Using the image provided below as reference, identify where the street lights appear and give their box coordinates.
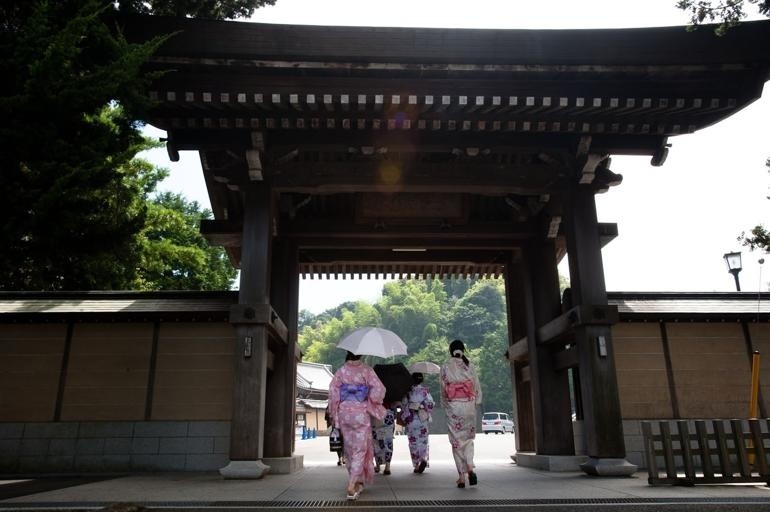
[723,251,743,291]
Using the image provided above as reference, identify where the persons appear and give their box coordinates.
[401,372,436,474]
[325,350,397,500]
[439,339,483,488]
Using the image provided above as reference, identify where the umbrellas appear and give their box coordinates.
[407,361,441,373]
[337,326,408,359]
[373,362,413,408]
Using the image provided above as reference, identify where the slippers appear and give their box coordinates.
[457,470,478,488]
[346,482,365,500]
[374,465,391,475]
[337,460,346,465]
[413,459,427,474]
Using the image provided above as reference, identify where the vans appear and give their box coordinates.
[481,412,515,434]
